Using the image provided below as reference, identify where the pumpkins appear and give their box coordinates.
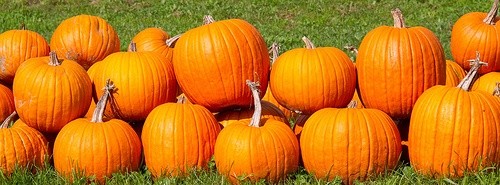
[0,0,500,185]
